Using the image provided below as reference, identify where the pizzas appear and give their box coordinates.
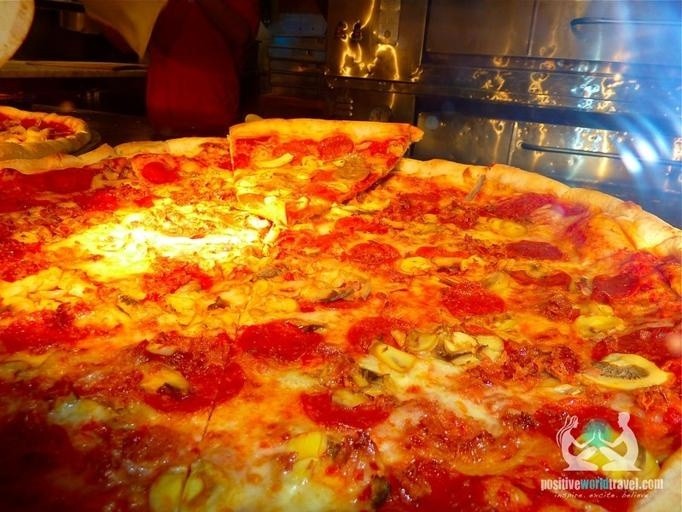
[0,117,682,511]
[0,105,92,162]
